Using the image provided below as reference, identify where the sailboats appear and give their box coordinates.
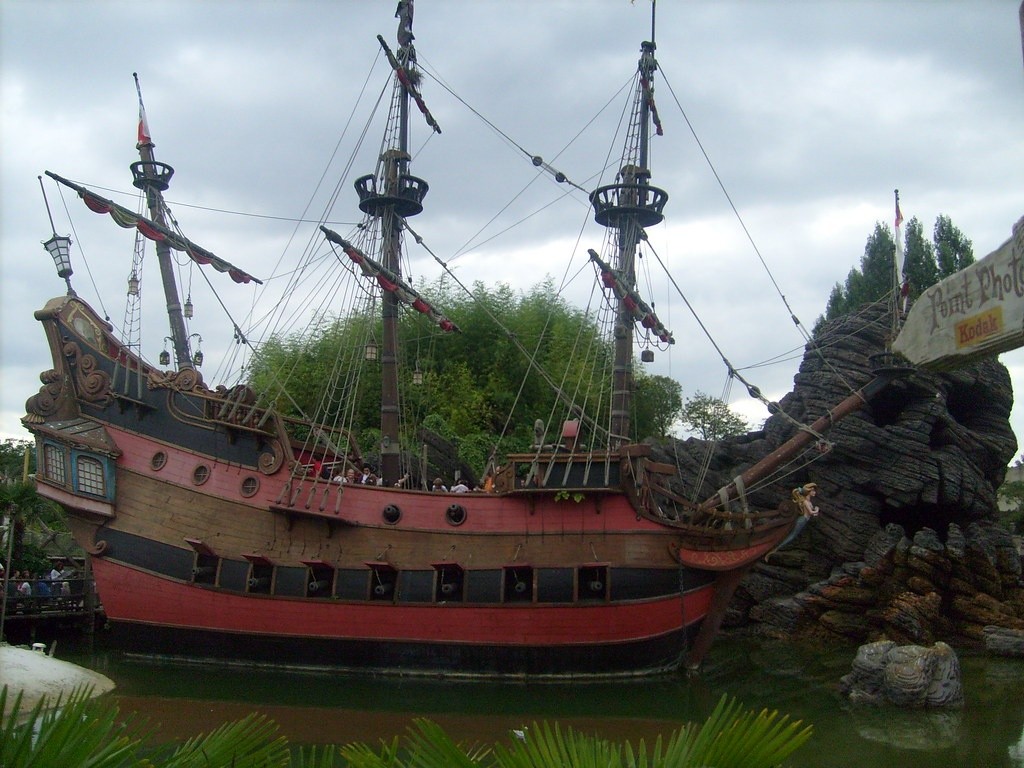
[18,1,917,690]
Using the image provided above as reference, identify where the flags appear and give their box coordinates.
[137,83,152,142]
[894,206,910,315]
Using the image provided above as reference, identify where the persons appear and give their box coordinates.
[309,454,324,477]
[333,467,347,482]
[346,468,358,484]
[8,561,72,604]
[432,478,448,492]
[357,462,377,485]
[450,478,471,493]
[765,483,820,562]
[485,473,493,491]
